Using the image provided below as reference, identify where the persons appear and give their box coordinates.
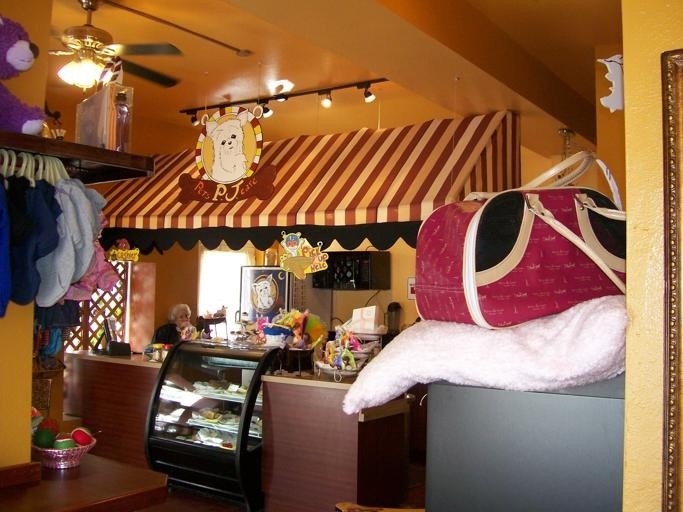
[150,303,195,347]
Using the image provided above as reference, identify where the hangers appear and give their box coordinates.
[1,147,72,193]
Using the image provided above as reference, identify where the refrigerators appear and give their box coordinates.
[235,264,332,335]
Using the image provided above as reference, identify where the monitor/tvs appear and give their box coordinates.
[269,348,314,375]
[90,318,113,353]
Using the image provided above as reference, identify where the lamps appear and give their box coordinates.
[179,73,385,126]
[57,52,114,90]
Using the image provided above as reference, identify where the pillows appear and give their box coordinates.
[341,290,641,418]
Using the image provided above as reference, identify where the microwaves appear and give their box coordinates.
[312,250,391,290]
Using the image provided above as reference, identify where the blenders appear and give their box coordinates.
[382,301,401,336]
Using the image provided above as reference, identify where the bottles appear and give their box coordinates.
[152,343,174,362]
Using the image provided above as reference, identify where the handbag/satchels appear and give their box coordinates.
[413,186,627,331]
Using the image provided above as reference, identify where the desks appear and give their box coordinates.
[0,442,170,511]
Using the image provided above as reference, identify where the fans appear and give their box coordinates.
[50,0,184,90]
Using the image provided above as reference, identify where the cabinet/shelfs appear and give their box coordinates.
[313,251,390,290]
[143,339,288,512]
